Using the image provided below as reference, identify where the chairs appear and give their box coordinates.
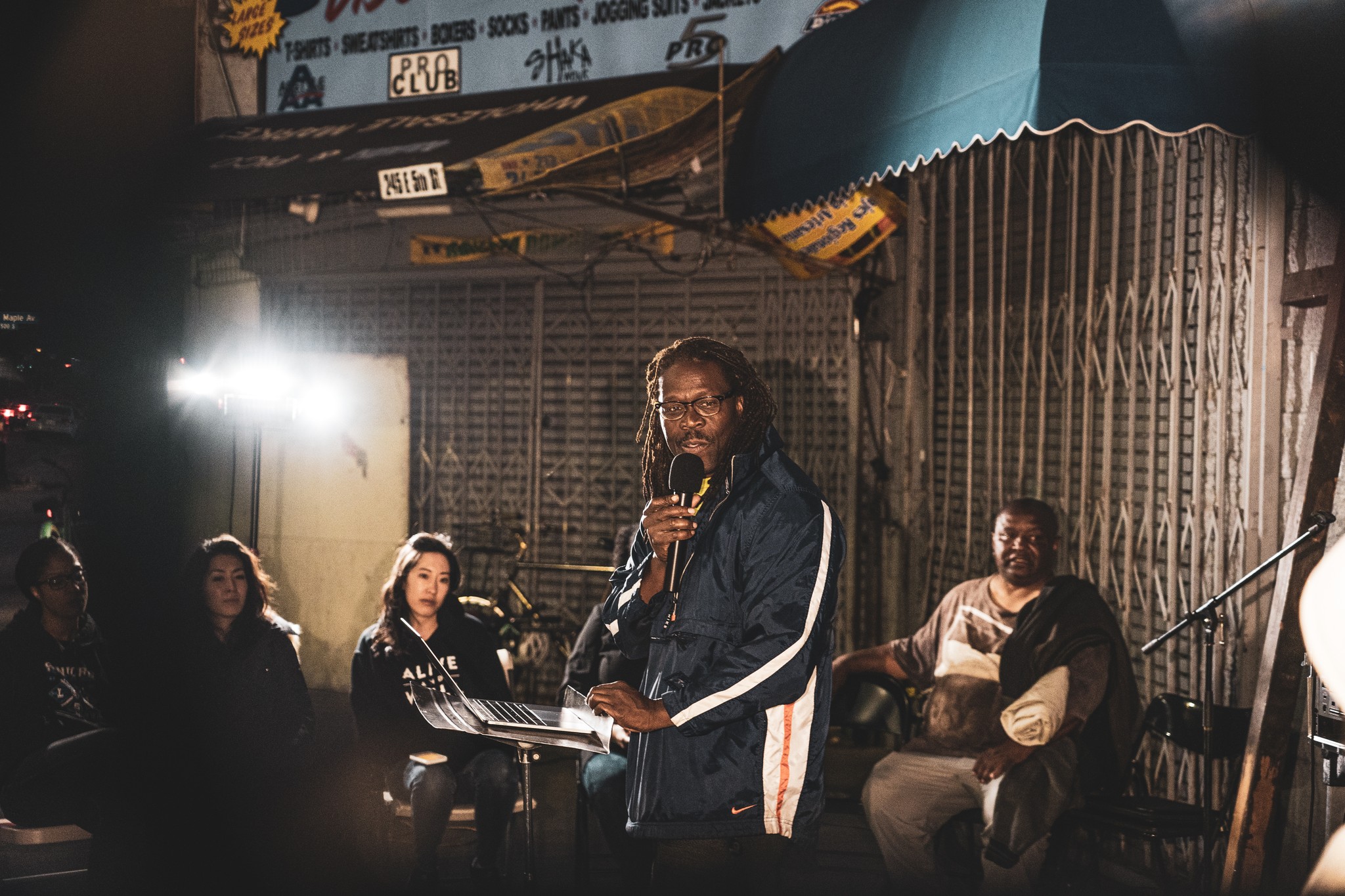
[1032,687,1262,896]
[732,671,915,896]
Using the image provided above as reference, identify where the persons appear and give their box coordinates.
[0,536,151,896]
[351,533,517,896]
[833,498,1110,896]
[558,522,658,896]
[173,533,318,896]
[584,337,847,896]
[1298,529,1345,896]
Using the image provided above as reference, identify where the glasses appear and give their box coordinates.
[42,569,86,591]
[655,389,737,420]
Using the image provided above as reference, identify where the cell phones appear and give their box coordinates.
[409,750,448,765]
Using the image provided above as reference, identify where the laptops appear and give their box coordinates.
[400,617,594,734]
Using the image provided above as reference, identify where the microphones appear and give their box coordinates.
[662,453,704,593]
